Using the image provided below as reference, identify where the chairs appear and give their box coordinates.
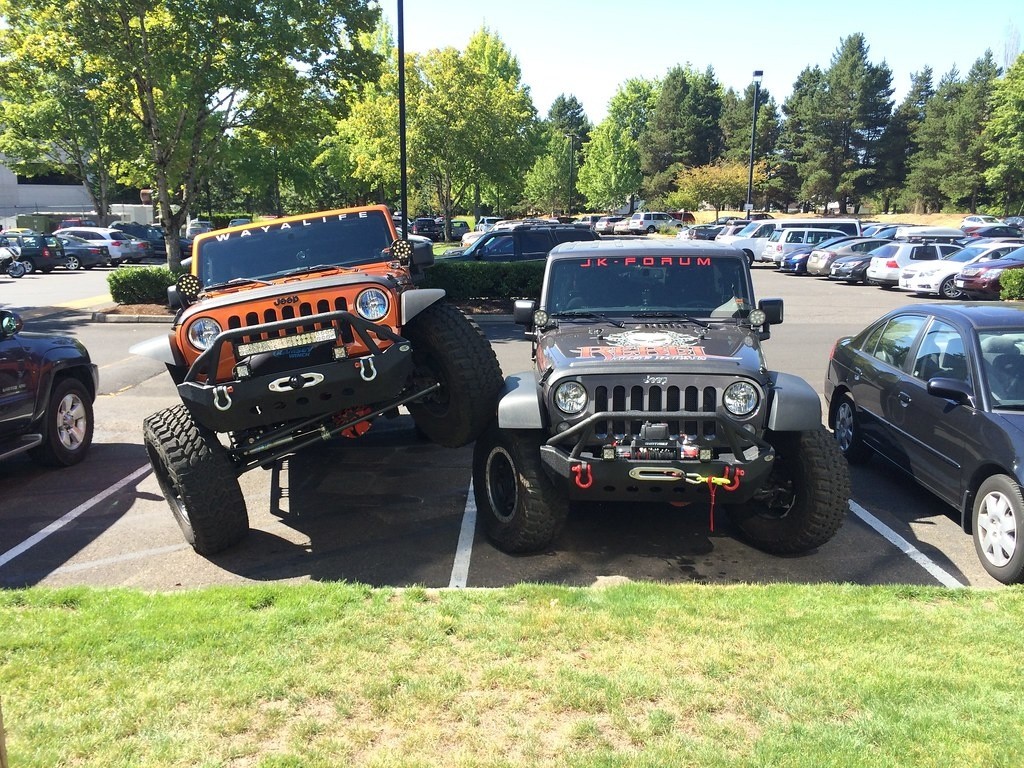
[812,236,820,244]
[991,252,1001,260]
[674,270,721,309]
[980,337,1020,372]
[793,238,802,243]
[931,338,1006,405]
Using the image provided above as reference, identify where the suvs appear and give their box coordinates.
[473,236,850,553]
[140,204,506,556]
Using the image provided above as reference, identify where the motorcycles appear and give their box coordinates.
[0,242,25,278]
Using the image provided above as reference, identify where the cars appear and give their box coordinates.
[825,304,1024,587]
[1,218,250,272]
[689,215,1024,299]
[392,210,696,255]
[0,310,99,469]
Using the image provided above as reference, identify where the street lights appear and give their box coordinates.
[746,69,764,203]
